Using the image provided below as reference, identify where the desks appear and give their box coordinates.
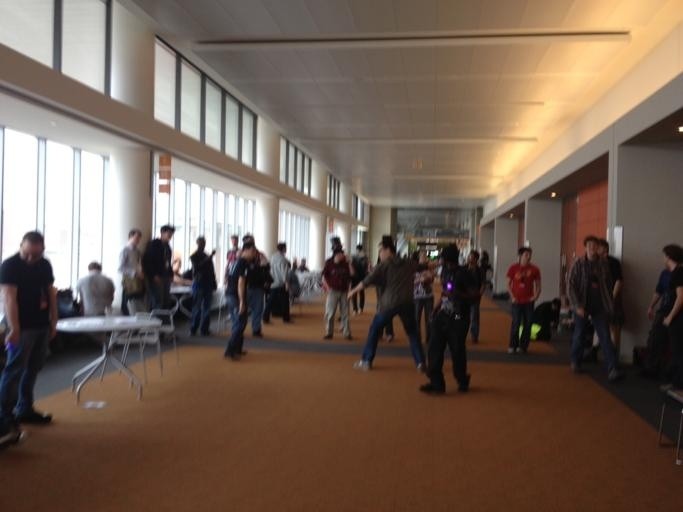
[295,271,324,297]
[169,287,193,319]
[55,316,163,404]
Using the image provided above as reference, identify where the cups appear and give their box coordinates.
[104,305,110,318]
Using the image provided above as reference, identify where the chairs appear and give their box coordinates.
[106,306,164,385]
[139,295,179,354]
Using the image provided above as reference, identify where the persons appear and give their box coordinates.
[75,263,115,316]
[645,244,683,393]
[505,247,541,354]
[564,238,622,384]
[0,316,28,447]
[3,231,58,434]
[118,225,309,360]
[322,235,489,393]
[532,298,561,342]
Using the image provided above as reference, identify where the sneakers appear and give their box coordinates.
[17,413,51,424]
[323,309,479,394]
[507,346,525,355]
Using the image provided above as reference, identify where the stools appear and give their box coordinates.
[657,385,683,466]
